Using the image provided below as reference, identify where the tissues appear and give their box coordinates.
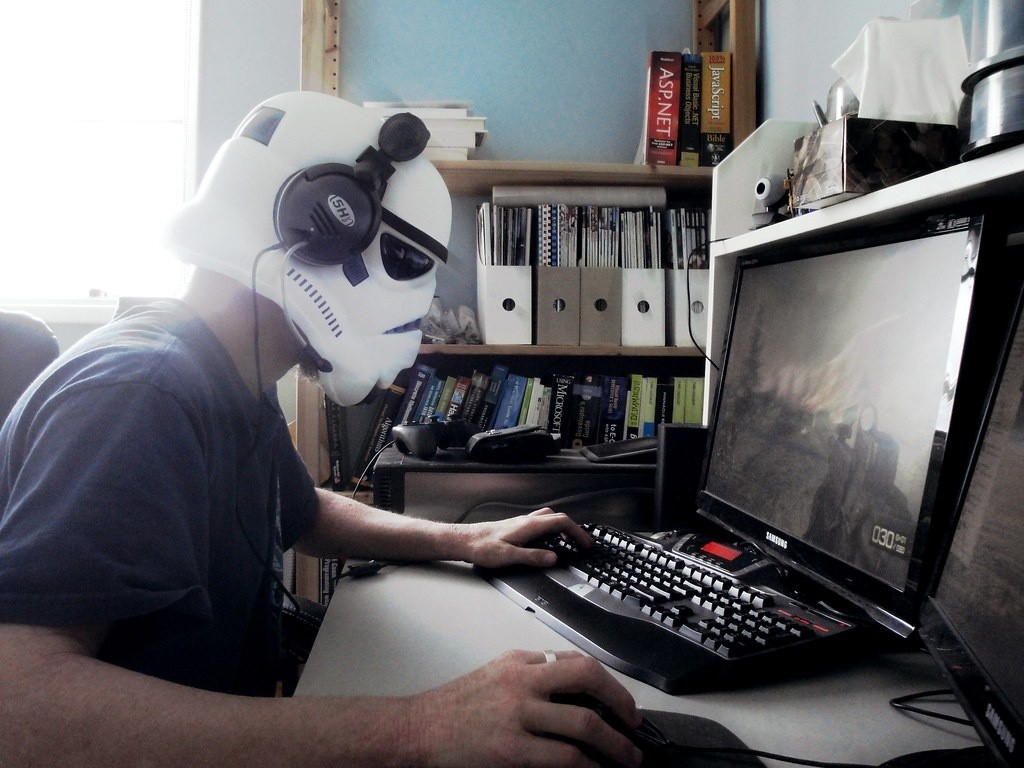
[793,15,969,211]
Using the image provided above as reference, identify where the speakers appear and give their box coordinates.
[654,422,710,547]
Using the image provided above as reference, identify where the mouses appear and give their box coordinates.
[530,682,670,768]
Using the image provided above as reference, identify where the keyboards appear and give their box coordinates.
[474,522,860,696]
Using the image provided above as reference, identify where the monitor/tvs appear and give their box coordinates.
[881,296,1024,768]
[701,168,1024,642]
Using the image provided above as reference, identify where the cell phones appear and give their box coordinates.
[581,436,658,461]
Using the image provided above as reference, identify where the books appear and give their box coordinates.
[323,361,706,495]
[475,200,711,267]
[317,555,341,608]
[644,49,732,169]
[358,95,487,161]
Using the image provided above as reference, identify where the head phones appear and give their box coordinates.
[273,113,432,267]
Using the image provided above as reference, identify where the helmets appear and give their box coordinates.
[174,91,451,406]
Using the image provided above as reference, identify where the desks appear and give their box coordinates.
[290,560,986,768]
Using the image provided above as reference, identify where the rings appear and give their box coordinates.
[541,650,558,665]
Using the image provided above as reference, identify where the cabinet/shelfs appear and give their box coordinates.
[294,0,756,606]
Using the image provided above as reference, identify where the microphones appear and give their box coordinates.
[281,241,335,374]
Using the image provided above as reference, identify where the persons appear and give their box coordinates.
[0,88,646,768]
